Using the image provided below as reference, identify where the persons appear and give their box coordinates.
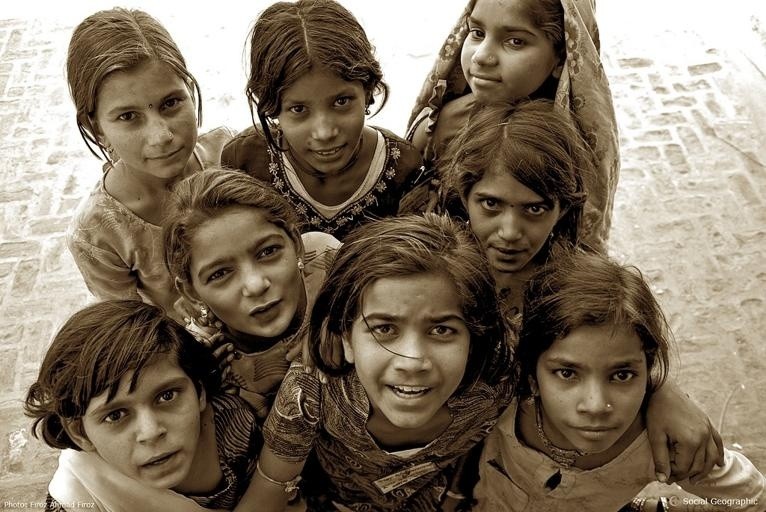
[435,93,601,383]
[463,235,766,510]
[169,0,431,329]
[63,4,288,388]
[229,209,729,511]
[20,295,321,512]
[398,0,624,264]
[159,167,354,430]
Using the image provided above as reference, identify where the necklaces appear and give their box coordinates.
[287,134,366,180]
[532,397,595,470]
